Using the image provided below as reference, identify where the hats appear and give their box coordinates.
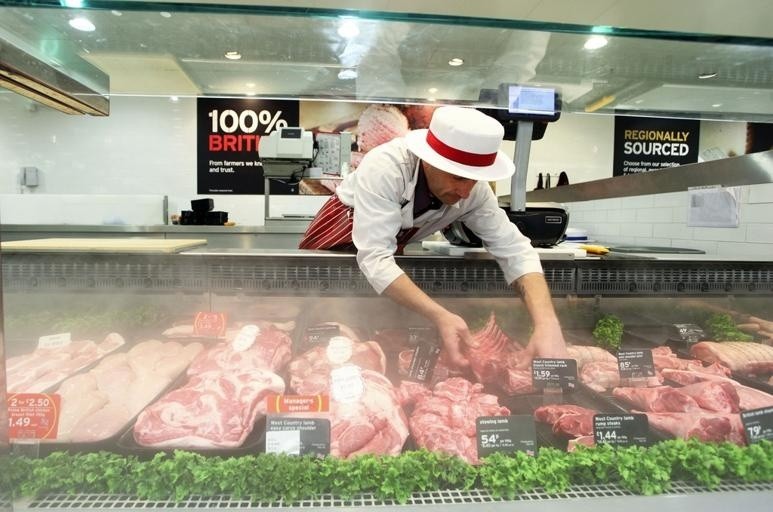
[401,105,516,182]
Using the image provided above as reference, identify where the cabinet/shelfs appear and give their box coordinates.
[0,246,773,512]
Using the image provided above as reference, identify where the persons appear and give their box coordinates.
[297,106,570,371]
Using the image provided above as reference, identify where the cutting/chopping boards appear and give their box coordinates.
[0,237,209,256]
